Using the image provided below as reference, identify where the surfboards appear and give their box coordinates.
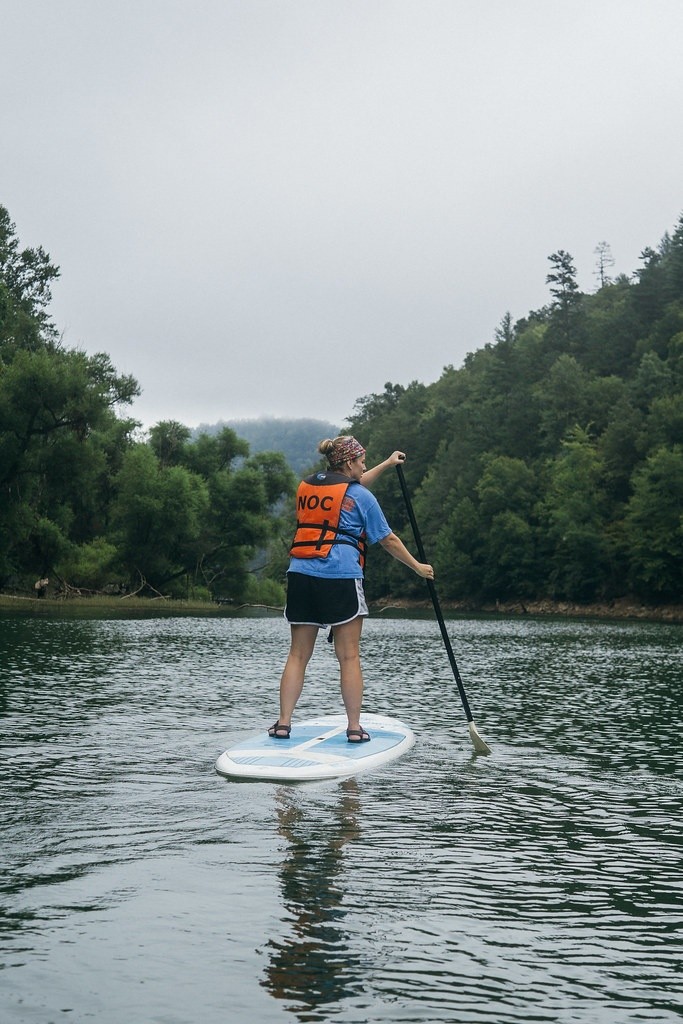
[212,710,418,782]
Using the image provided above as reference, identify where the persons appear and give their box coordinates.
[267,436,434,743]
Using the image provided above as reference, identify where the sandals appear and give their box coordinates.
[346,725,370,743]
[268,720,292,738]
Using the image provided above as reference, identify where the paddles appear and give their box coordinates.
[394,454,495,753]
